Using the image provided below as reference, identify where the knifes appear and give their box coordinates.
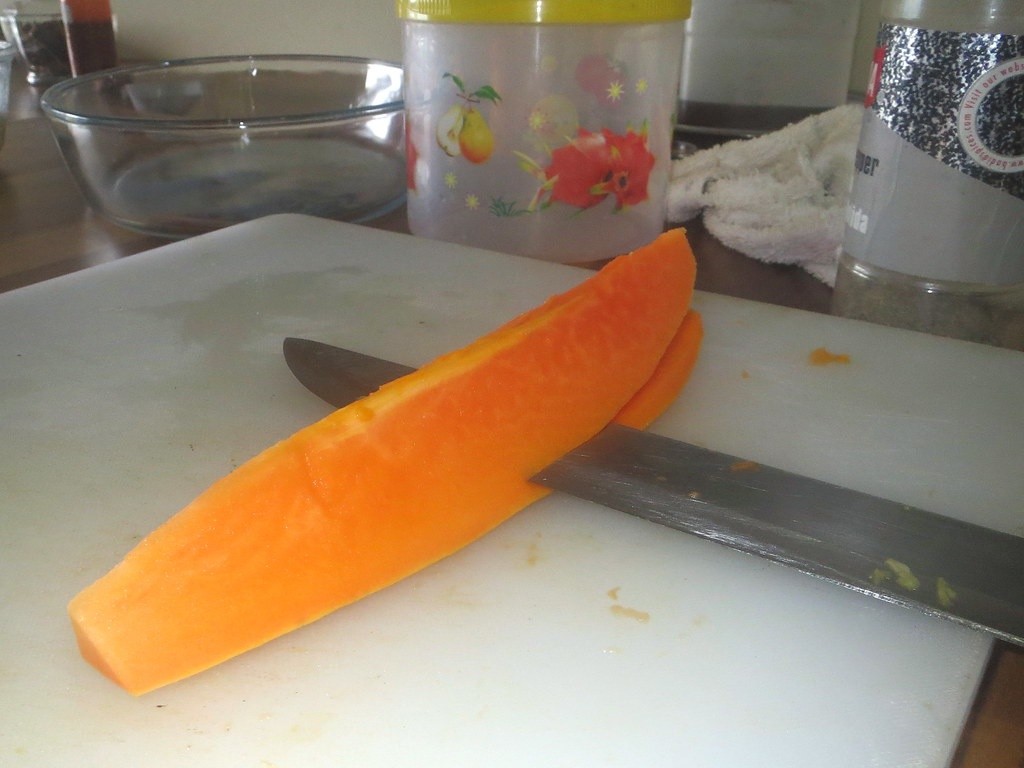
[284,337,1024,650]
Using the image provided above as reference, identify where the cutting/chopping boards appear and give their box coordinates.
[1,211,1024,768]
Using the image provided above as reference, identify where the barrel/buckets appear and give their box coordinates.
[399,0,694,264]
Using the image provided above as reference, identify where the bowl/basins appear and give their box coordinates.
[0,8,119,86]
[41,51,404,239]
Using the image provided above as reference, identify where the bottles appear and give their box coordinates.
[61,0,118,80]
[830,1,1024,346]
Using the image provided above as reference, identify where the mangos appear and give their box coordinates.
[69,224,702,699]
